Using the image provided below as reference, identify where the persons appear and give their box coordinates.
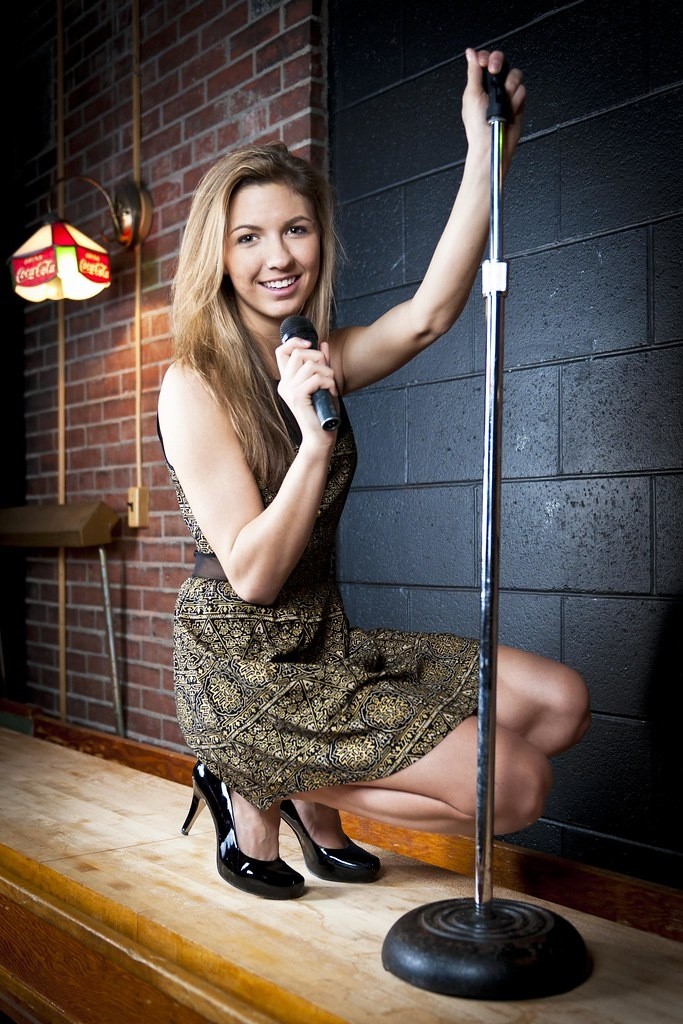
[155,42,591,901]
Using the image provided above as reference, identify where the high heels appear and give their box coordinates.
[280,799,381,883]
[182,760,305,900]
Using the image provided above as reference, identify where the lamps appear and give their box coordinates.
[12,174,154,302]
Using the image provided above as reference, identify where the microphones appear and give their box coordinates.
[280,315,340,432]
